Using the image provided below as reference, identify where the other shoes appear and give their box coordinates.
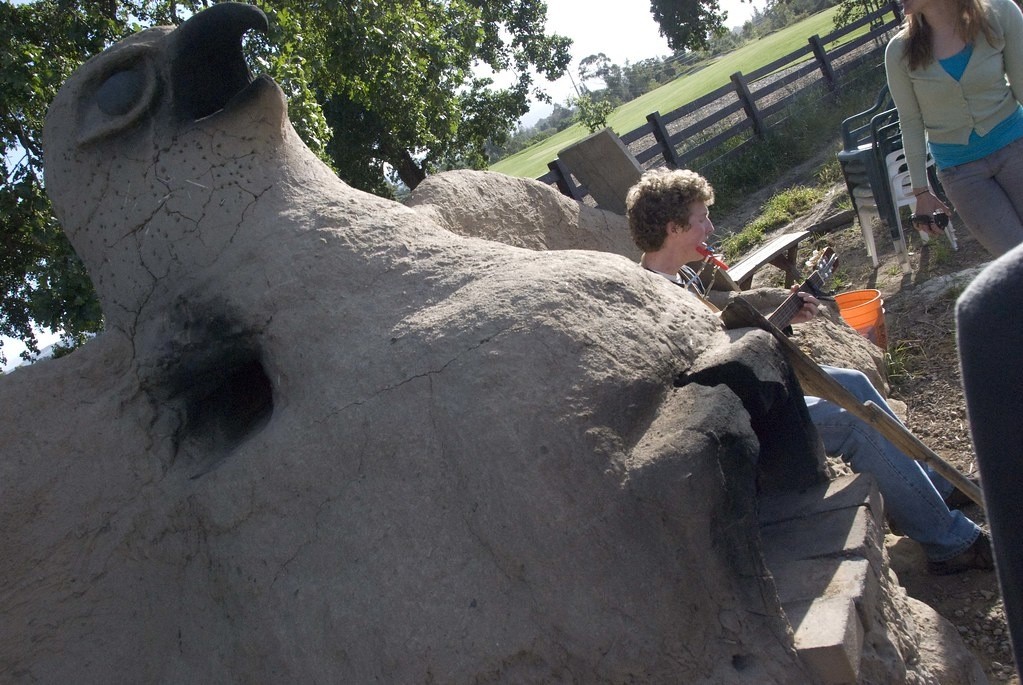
[942,476,982,512]
[931,530,996,573]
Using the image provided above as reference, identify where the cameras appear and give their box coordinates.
[933,212,949,230]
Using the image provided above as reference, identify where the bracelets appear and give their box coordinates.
[915,190,929,196]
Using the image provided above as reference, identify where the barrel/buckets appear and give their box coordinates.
[833,289,888,353]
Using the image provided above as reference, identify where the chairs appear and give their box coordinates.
[838,82,959,274]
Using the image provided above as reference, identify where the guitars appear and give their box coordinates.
[767,246,841,332]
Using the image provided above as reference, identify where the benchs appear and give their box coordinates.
[725,230,811,292]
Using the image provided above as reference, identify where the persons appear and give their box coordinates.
[885,0,1023,258]
[626,167,992,576]
